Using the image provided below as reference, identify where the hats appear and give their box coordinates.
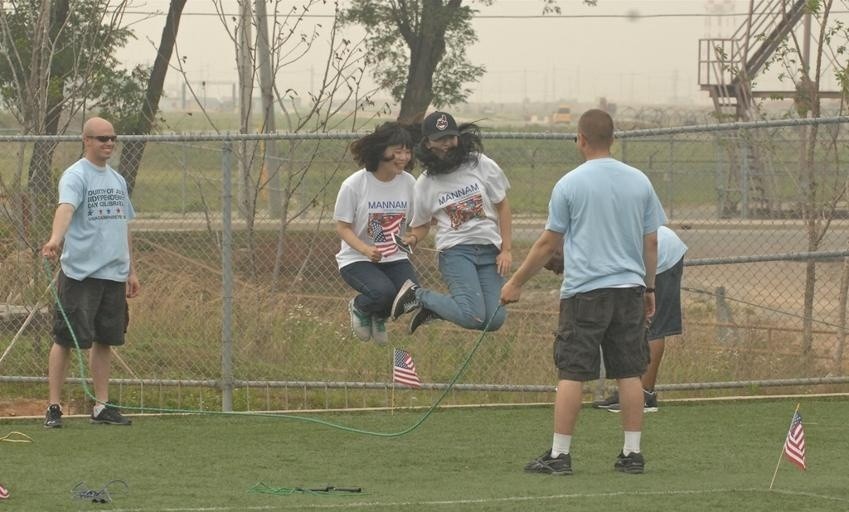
[421,111,461,140]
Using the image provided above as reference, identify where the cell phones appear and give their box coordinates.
[391,233,413,255]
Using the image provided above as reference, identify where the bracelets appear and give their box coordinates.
[644,287,656,293]
[407,234,418,245]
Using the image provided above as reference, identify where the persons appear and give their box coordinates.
[390,112,513,334]
[541,223,689,412]
[334,122,420,345]
[42,117,142,428]
[499,108,667,475]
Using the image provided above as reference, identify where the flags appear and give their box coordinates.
[370,216,402,257]
[394,347,421,390]
[783,409,808,470]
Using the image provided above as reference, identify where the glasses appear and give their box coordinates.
[87,136,116,142]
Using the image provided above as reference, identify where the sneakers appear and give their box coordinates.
[592,392,621,409]
[524,449,573,476]
[89,402,131,425]
[348,280,433,345]
[46,404,64,426]
[608,389,658,413]
[614,449,645,473]
